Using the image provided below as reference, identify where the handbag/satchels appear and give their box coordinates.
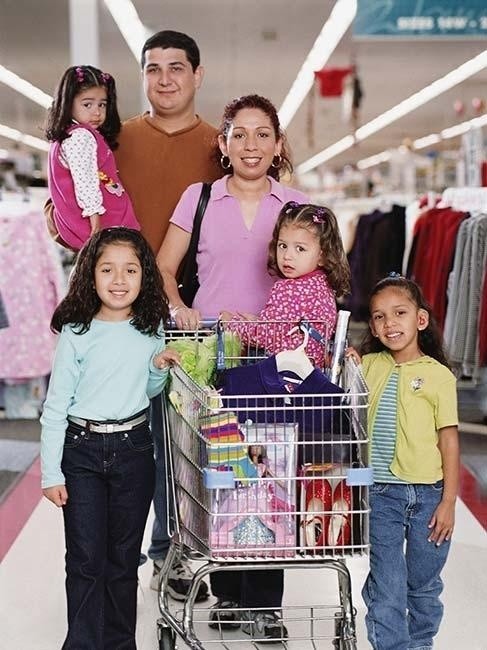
[174,245,200,310]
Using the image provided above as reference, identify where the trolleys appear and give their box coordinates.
[155,317,371,650]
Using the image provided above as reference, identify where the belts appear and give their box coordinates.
[67,412,149,436]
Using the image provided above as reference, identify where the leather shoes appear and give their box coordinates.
[303,475,333,551]
[325,478,357,546]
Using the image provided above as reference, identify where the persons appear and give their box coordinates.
[344,274,462,649]
[45,64,144,248]
[42,28,229,602]
[214,199,352,396]
[153,92,306,647]
[37,225,181,650]
[214,439,288,544]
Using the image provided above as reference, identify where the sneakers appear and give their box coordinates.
[149,555,210,603]
[207,598,244,630]
[240,610,289,644]
